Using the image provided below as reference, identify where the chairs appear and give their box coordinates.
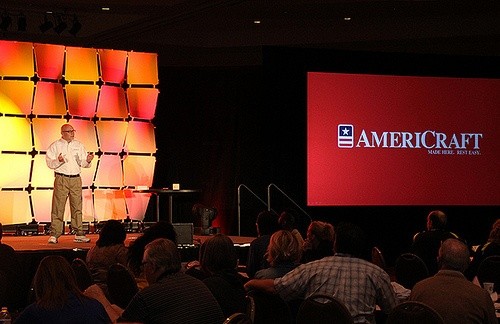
[73,246,500,324]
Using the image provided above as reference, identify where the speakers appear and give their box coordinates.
[171,223,194,244]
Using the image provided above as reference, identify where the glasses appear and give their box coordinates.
[62,130,76,134]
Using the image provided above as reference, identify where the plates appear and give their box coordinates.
[494,303,500,309]
[495,313,500,317]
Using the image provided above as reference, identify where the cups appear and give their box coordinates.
[173,184,179,190]
[483,283,493,293]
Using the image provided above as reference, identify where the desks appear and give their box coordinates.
[133,189,202,223]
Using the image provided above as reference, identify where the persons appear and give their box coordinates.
[0,209,500,324]
[45,123,94,243]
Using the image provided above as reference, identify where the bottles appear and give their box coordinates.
[0,307,11,324]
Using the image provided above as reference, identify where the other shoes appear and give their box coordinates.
[74,234,91,242]
[48,236,58,243]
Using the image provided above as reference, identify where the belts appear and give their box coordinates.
[55,172,80,178]
